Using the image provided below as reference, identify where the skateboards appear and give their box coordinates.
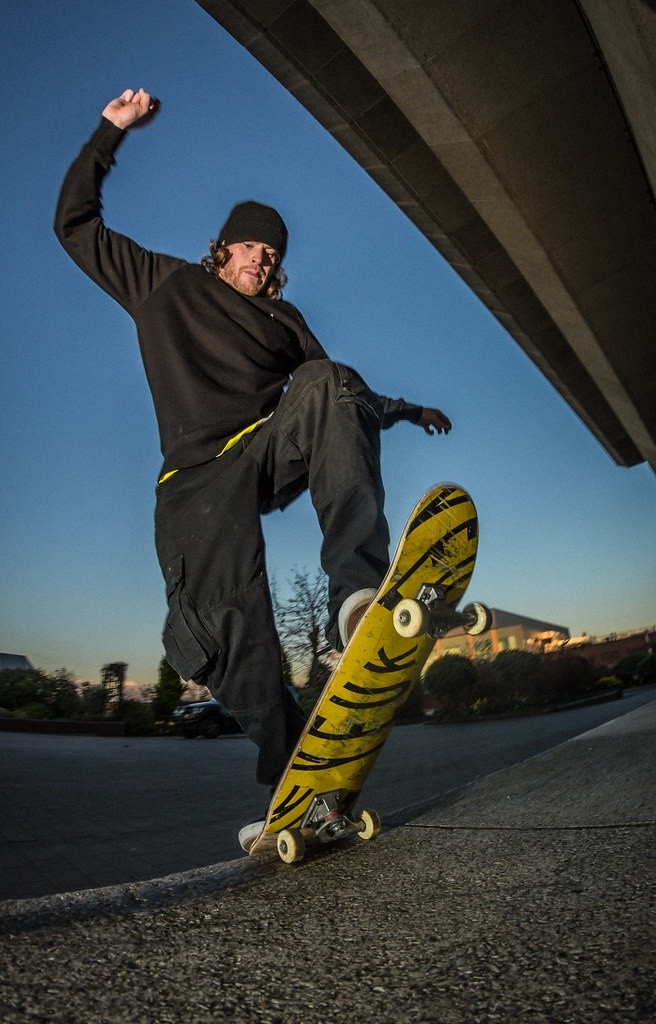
[248,481,494,865]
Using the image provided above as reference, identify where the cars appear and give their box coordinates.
[173,682,300,741]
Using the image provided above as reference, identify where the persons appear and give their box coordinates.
[54,85,452,854]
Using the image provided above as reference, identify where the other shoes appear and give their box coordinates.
[237,816,265,852]
[336,587,378,648]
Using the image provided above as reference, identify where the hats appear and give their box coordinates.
[216,201,288,264]
